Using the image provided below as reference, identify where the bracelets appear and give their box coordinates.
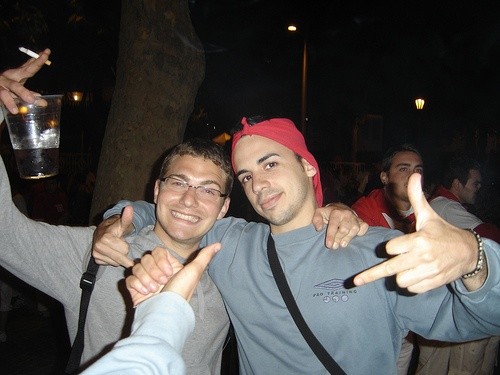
[462,228,484,279]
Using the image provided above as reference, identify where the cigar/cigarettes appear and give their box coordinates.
[19,47,51,66]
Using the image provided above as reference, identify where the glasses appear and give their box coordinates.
[160,177,228,202]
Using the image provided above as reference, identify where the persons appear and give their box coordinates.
[0,48,368,375]
[78,242,223,375]
[349,142,500,375]
[91,119,500,375]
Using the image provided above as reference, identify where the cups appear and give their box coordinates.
[2,94,62,179]
